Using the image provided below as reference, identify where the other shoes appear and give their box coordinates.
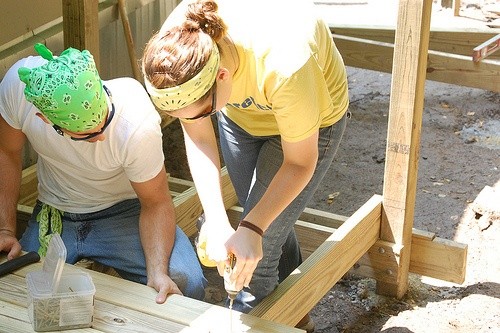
[297,320,315,333]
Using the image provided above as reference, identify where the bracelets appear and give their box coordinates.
[239,221,265,236]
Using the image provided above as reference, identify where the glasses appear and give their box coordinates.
[39,83,116,141]
[184,80,217,120]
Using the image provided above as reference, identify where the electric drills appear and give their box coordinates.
[195,214,244,331]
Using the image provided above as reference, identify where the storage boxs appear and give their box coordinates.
[25,231,95,332]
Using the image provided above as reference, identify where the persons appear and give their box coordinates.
[1,43,208,304]
[140,0,350,333]
